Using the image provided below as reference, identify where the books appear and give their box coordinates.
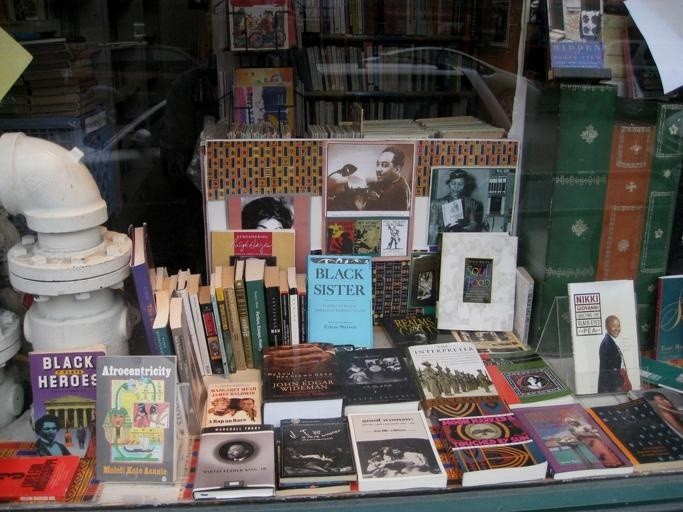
[0,0,683,506]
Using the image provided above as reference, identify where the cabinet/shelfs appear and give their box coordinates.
[208,0,306,139]
[292,1,490,122]
[0,350,682,512]
[0,0,155,221]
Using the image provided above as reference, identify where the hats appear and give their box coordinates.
[446,169,467,184]
